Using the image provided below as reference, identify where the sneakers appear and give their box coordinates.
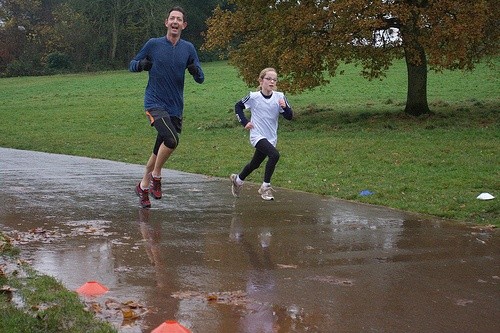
[135,182,151,208]
[147,172,161,199]
[258,185,277,201]
[230,174,244,198]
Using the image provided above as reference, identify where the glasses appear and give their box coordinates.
[264,78,277,82]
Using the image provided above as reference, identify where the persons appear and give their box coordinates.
[128,5,205,208]
[229,68,293,200]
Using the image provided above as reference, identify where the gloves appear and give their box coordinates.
[188,64,198,76]
[139,57,153,71]
